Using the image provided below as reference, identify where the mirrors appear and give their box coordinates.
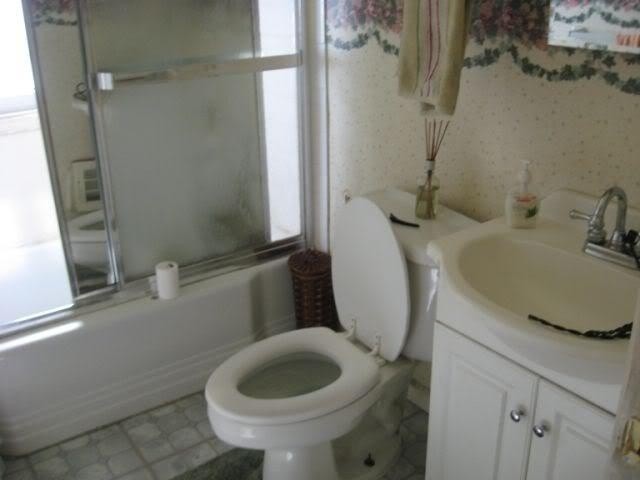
[20,0,120,304]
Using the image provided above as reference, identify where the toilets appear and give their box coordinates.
[202,187,482,480]
[67,208,109,270]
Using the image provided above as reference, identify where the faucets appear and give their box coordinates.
[585,185,629,248]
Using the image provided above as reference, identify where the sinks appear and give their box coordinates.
[424,187,640,387]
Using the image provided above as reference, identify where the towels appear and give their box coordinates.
[398,2,473,118]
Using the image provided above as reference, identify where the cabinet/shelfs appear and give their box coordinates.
[424,323,639,480]
